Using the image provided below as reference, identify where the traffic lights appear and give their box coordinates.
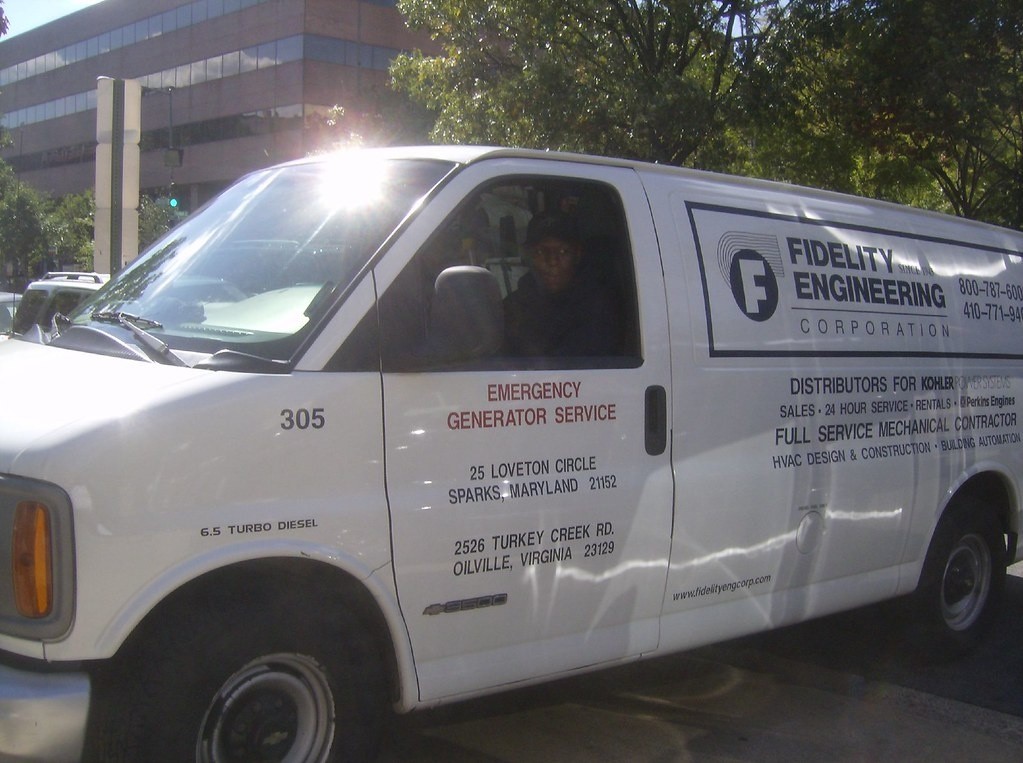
[168,182,181,208]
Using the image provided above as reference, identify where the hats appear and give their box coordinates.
[520,209,580,248]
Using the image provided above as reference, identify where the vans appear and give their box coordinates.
[1,140,1022,763]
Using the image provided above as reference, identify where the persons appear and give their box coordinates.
[491,197,624,359]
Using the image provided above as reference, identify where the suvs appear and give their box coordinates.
[0,267,114,346]
